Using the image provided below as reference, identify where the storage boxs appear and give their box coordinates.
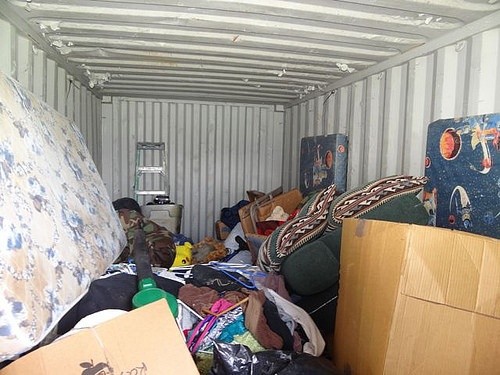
[0,297,200,375]
[333,218,500,375]
[141,204,183,234]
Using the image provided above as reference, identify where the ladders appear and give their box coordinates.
[132,141,172,202]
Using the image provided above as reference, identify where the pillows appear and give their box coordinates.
[258,183,337,274]
[325,174,430,235]
